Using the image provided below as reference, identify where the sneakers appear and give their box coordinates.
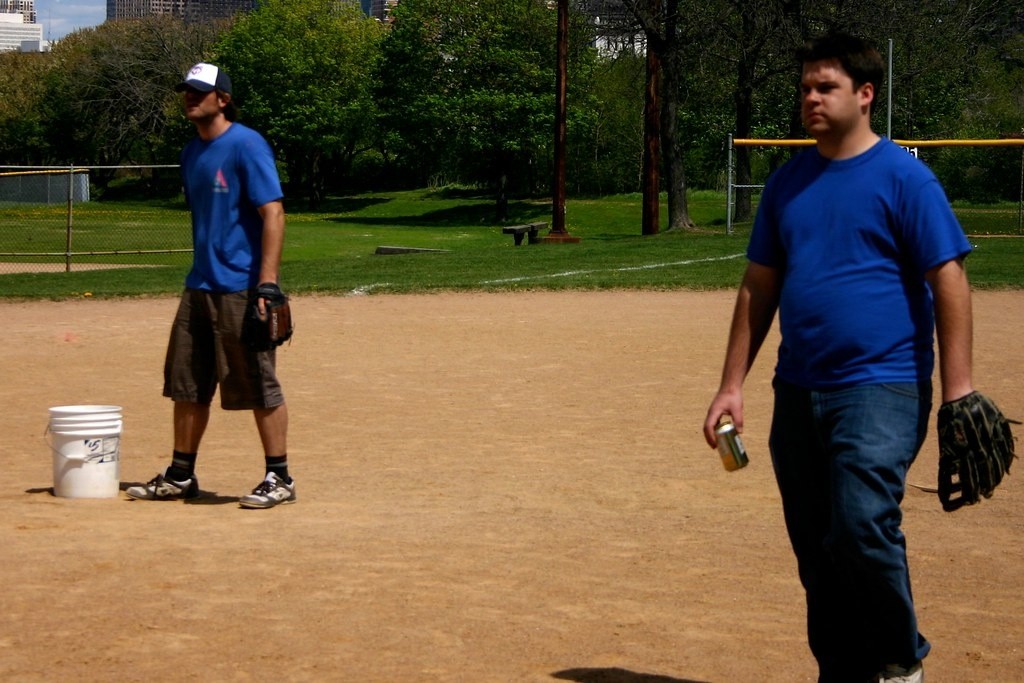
[879,660,924,683]
[240,471,296,508]
[126,474,200,499]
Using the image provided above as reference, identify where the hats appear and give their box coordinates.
[174,62,233,96]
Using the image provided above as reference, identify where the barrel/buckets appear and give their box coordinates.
[43,405,123,498]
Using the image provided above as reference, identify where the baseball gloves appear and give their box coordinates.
[254,282,293,351]
[937,390,1022,513]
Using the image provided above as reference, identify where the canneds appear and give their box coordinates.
[715,421,748,471]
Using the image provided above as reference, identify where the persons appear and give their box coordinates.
[124,61,297,509]
[704,31,981,683]
